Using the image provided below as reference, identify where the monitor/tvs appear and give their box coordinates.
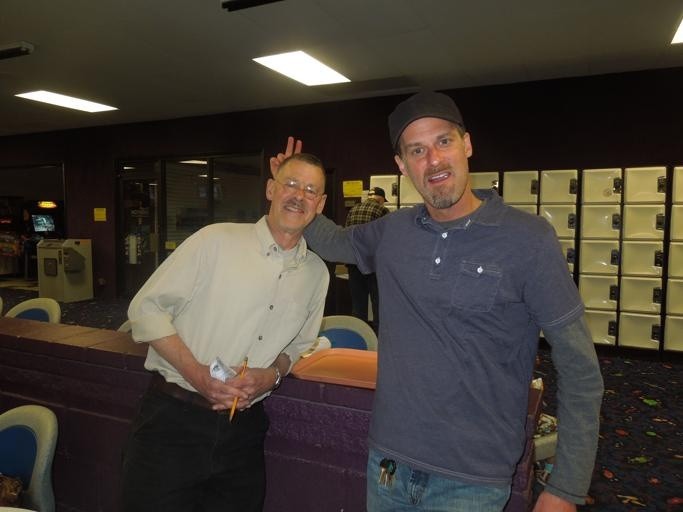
[31,214,57,233]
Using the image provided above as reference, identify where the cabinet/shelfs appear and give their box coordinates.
[369,174,427,213]
[467,171,539,216]
[536,166,683,353]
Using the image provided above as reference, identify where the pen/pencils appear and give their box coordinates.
[228,356,247,420]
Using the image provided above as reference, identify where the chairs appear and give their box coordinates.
[0,404,60,512]
[4,296,61,324]
[320,314,379,353]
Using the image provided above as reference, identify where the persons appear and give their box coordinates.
[123,151,331,511]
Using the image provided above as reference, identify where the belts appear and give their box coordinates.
[152,378,213,409]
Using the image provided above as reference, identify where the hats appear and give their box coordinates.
[368,187,388,202]
[388,91,463,149]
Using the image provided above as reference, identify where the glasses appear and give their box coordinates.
[273,177,318,195]
